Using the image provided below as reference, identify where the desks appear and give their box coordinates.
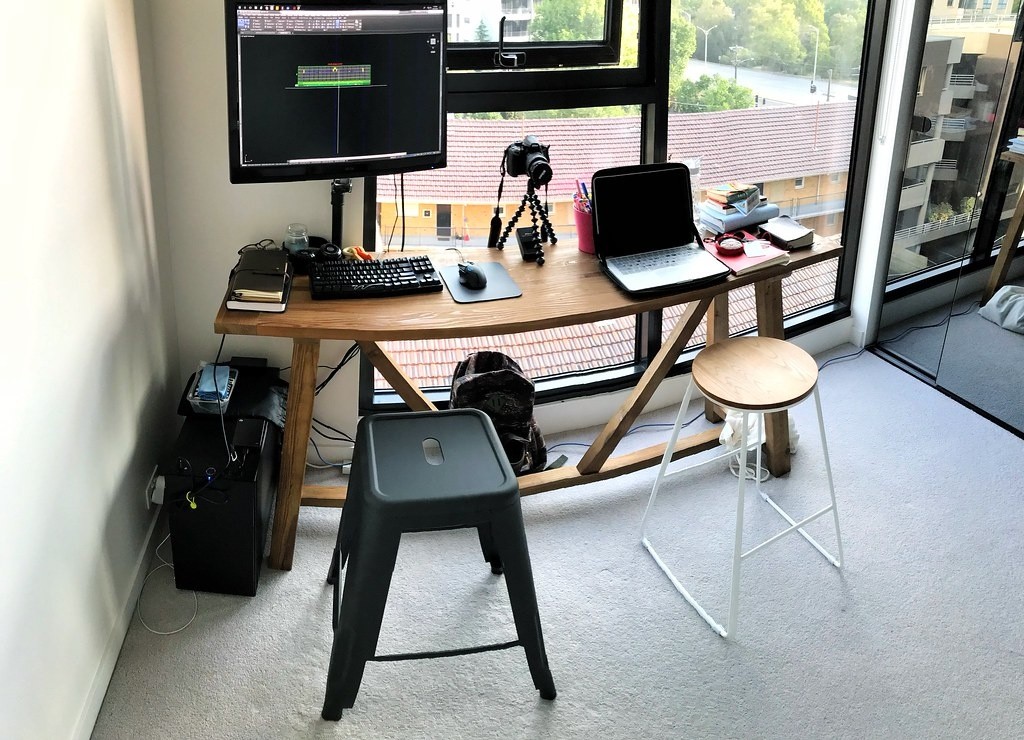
[216,230,845,572]
[980,151,1024,307]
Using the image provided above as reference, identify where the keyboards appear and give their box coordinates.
[308,254,443,301]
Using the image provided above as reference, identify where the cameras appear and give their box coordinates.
[506,137,552,186]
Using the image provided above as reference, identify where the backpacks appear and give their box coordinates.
[453,351,568,477]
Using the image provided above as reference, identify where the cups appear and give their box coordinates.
[573,205,595,254]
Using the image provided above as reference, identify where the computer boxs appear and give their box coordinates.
[166,367,283,597]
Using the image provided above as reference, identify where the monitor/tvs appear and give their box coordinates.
[225,0,447,184]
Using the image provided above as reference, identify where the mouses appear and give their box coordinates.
[457,260,487,291]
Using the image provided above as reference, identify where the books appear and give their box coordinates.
[698,181,779,236]
[1006,126,1024,155]
[224,250,294,314]
[757,216,814,251]
[701,230,791,277]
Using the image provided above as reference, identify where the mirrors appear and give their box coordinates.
[876,0,1024,436]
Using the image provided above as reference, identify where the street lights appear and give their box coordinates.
[807,24,819,93]
[729,45,754,82]
[696,25,718,75]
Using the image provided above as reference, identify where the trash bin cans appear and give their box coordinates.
[451,351,535,476]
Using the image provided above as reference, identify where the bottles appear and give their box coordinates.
[683,159,701,226]
[285,223,309,255]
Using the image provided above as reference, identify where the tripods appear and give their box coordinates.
[497,179,558,266]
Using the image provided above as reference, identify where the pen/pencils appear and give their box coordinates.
[575,180,581,194]
[574,195,583,211]
[581,182,592,205]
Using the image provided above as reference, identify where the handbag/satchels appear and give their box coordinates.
[720,410,801,483]
[978,285,1024,334]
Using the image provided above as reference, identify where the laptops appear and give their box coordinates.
[591,166,730,292]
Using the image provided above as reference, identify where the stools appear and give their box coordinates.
[322,408,557,722]
[636,337,845,639]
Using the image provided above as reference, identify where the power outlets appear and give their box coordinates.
[145,464,160,509]
